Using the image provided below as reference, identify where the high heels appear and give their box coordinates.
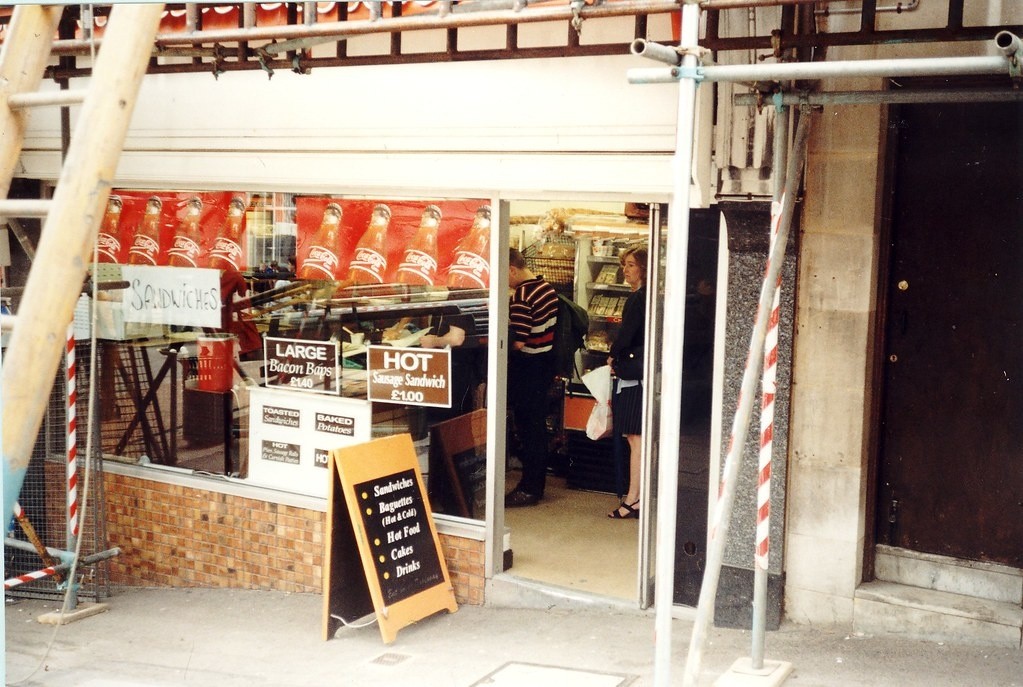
[607,498,639,519]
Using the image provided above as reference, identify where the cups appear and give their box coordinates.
[349,333,364,345]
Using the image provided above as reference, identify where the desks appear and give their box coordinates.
[109,332,197,464]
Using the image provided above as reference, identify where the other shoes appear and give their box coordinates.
[505,487,539,508]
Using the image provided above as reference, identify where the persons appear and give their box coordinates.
[505,247,558,508]
[608,246,663,518]
[387,314,482,517]
[254,257,296,314]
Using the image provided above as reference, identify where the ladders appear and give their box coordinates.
[0,4,163,540]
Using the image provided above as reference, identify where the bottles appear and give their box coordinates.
[300,202,491,290]
[89,193,248,268]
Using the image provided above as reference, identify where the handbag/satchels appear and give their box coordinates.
[580,364,614,442]
[615,345,643,380]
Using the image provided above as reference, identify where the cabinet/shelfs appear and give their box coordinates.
[572,238,647,385]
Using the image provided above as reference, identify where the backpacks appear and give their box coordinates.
[548,291,590,378]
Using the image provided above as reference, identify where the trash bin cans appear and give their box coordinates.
[176,356,235,445]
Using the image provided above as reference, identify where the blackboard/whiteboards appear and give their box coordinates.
[333,431,455,631]
